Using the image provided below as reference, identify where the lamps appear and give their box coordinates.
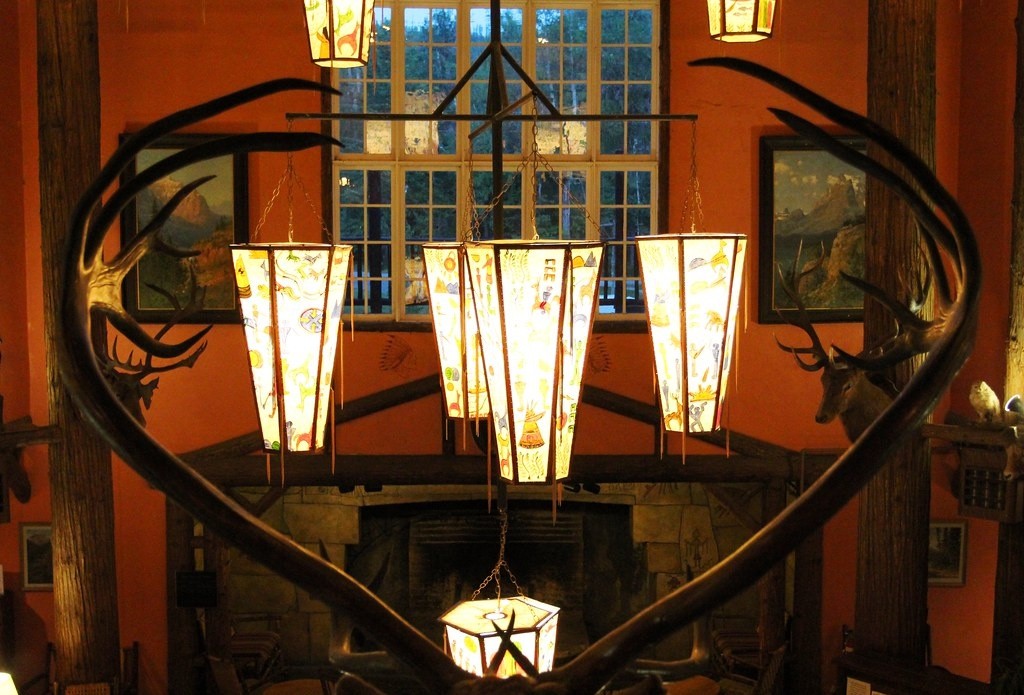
[229,117,354,488]
[707,0,778,44]
[634,120,747,465]
[461,95,610,526]
[435,510,563,679]
[300,0,374,69]
[420,141,492,440]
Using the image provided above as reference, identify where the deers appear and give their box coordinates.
[52,55,983,695]
[101,259,216,431]
[771,237,947,445]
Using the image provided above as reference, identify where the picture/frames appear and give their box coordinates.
[116,130,248,325]
[758,134,864,324]
[927,519,968,589]
[17,521,53,592]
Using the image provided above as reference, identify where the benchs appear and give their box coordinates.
[708,614,758,678]
[225,616,286,695]
[18,641,139,695]
[717,649,784,695]
